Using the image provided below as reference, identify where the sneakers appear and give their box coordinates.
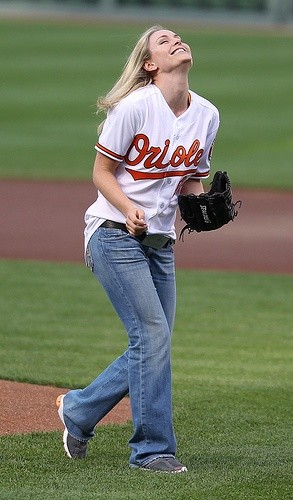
[131,457,188,473]
[56,393,87,459]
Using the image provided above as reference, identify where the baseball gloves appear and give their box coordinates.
[176,170,237,232]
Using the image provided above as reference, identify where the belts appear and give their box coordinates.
[99,219,175,250]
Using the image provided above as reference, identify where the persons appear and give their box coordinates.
[56,23,221,473]
